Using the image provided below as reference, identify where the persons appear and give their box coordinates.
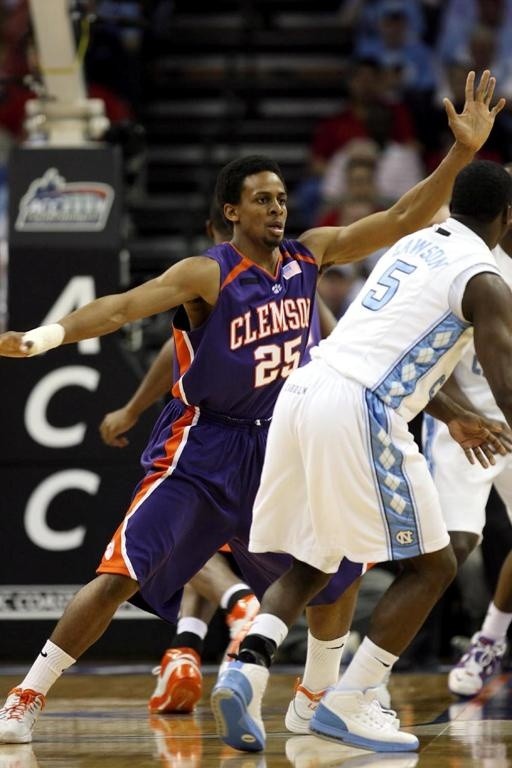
[1,70,512,757]
[307,0,512,334]
[0,0,171,171]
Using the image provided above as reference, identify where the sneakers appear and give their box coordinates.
[0,685,47,743]
[209,659,270,752]
[147,648,204,714]
[449,631,509,699]
[284,677,331,735]
[221,597,260,671]
[308,684,419,752]
